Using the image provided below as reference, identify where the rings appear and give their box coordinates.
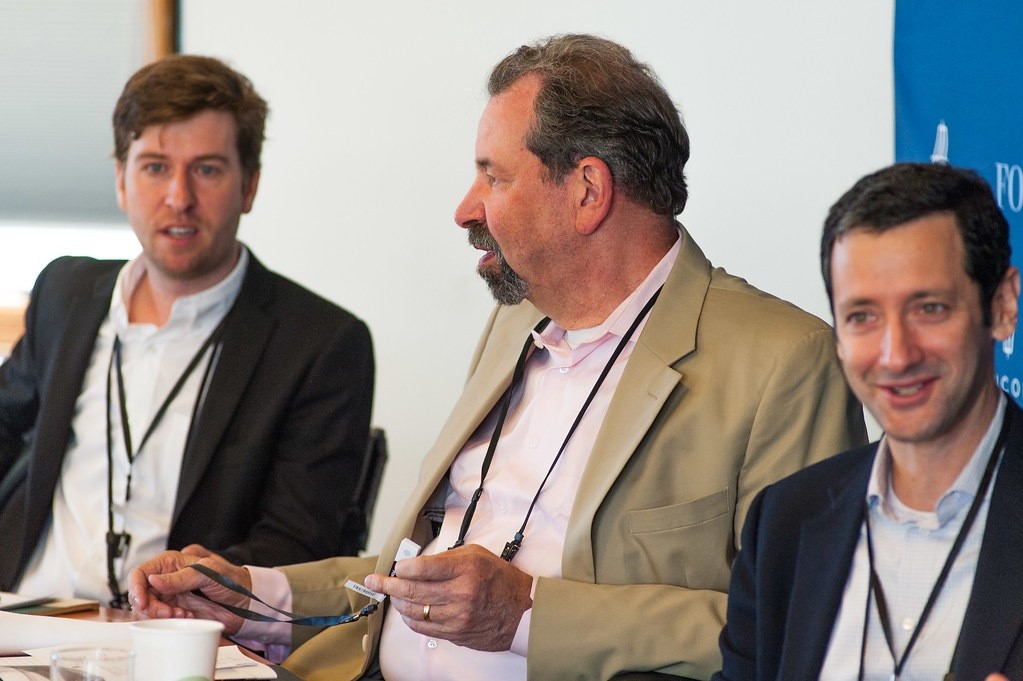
[422,604,432,623]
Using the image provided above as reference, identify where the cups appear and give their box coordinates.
[130,618,225,680]
[50,646,135,680]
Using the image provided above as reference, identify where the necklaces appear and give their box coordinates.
[856,390,1009,678]
[444,282,663,562]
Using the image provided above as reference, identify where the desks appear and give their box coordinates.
[0,607,305,681]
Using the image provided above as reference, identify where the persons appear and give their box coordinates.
[0,55,375,613]
[698,162,1021,680]
[126,32,849,681]
